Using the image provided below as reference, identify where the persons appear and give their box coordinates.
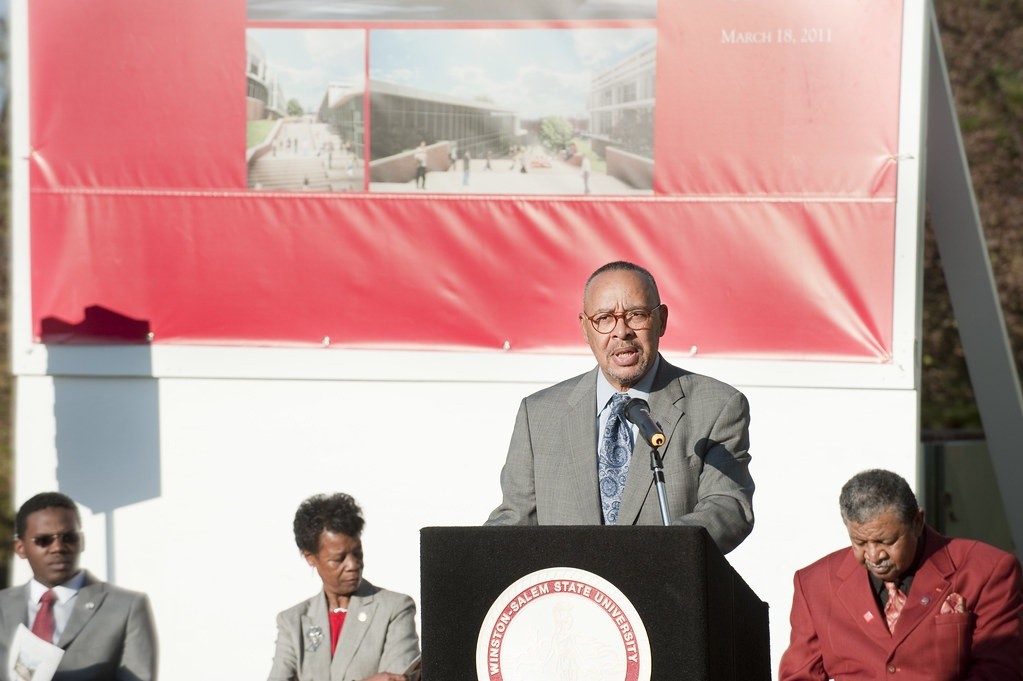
[272,138,358,191]
[415,142,591,192]
[778,469,1023,681]
[0,492,156,681]
[482,262,756,553]
[265,493,421,681]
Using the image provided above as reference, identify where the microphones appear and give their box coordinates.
[623,397,665,448]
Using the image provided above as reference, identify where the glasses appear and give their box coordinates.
[584,304,660,334]
[19,528,82,547]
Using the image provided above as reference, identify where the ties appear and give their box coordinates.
[31,589,57,644]
[885,581,907,636]
[598,393,635,525]
